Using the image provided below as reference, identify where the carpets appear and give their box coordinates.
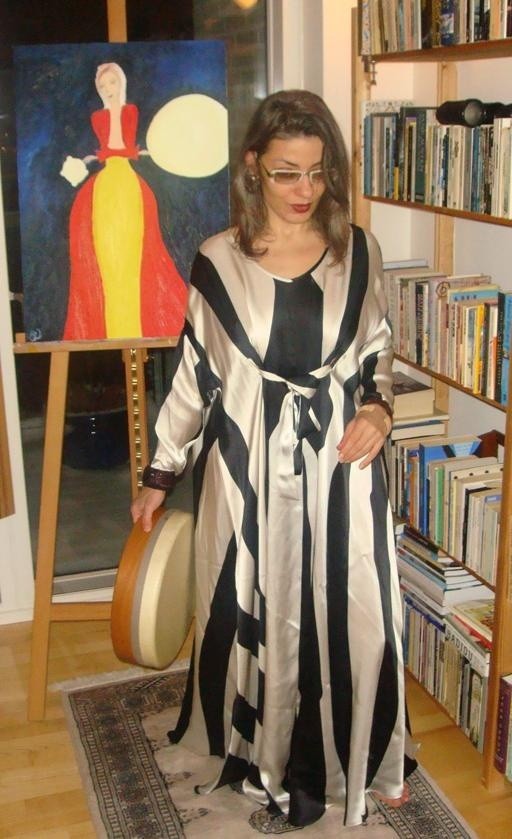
[47,657,478,837]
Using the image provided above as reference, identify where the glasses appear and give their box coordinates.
[256,158,327,186]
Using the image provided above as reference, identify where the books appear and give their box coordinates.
[389,409,505,585]
[360,98,512,220]
[396,555,512,785]
[385,258,511,407]
[358,1,512,55]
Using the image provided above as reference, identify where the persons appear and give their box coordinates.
[130,90,413,831]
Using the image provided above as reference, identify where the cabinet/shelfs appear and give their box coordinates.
[349,3,512,794]
